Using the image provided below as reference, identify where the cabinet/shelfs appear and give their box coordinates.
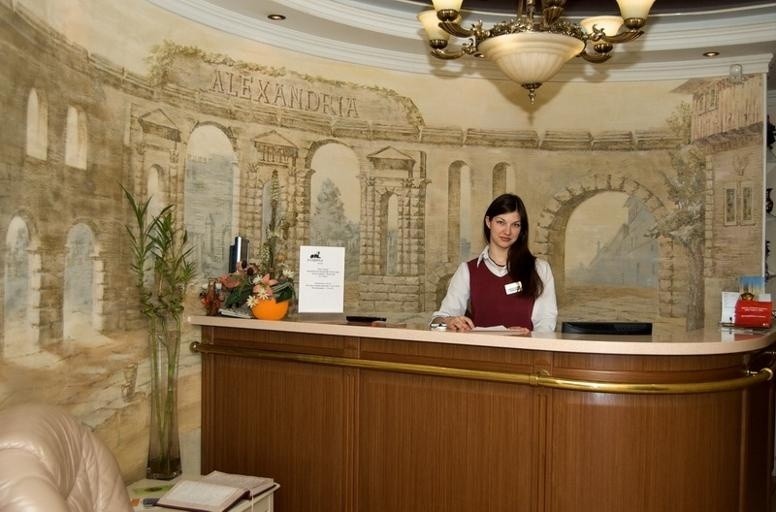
[126,475,280,511]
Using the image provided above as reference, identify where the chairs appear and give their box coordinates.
[563,322,652,336]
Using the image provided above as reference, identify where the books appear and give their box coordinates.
[154,471,280,511]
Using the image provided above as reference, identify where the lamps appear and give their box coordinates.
[415,1,653,98]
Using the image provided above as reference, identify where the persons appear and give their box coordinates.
[430,193,558,336]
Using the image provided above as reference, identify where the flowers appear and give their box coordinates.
[226,169,298,307]
[119,183,198,482]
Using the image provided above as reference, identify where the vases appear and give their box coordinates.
[144,328,184,481]
[249,294,290,319]
